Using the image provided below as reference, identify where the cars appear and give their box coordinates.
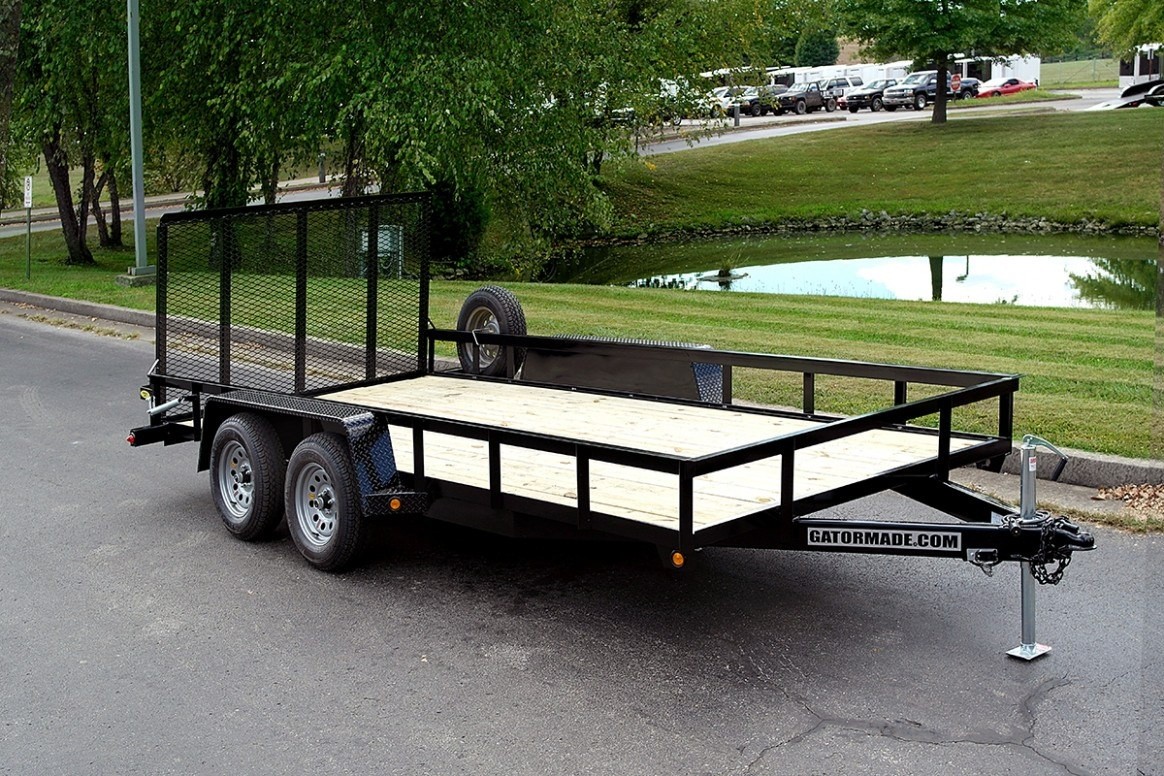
[586,54,1046,128]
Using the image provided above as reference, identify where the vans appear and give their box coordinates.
[1116,42,1164,107]
